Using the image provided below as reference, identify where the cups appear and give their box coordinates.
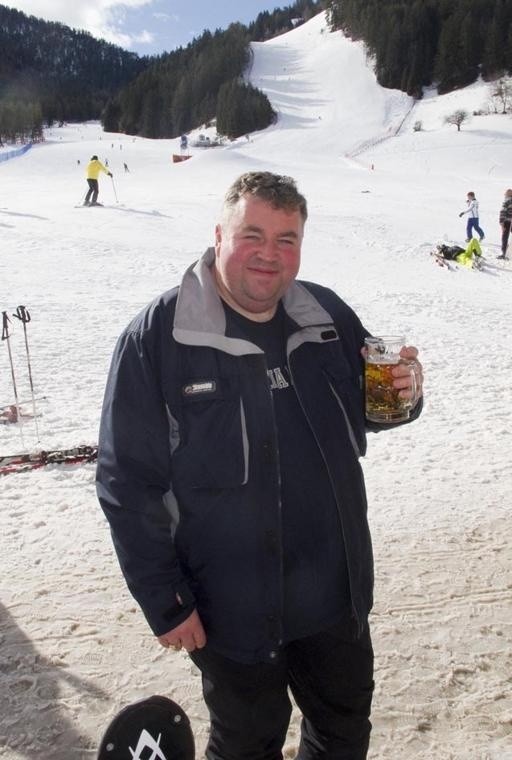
[363,335,421,425]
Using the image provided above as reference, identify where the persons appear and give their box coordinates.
[103,158,110,167]
[91,170,425,759]
[434,238,485,272]
[83,155,112,207]
[496,189,512,259]
[458,191,485,243]
[122,162,130,172]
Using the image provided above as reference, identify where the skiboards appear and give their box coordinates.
[0,443,98,474]
